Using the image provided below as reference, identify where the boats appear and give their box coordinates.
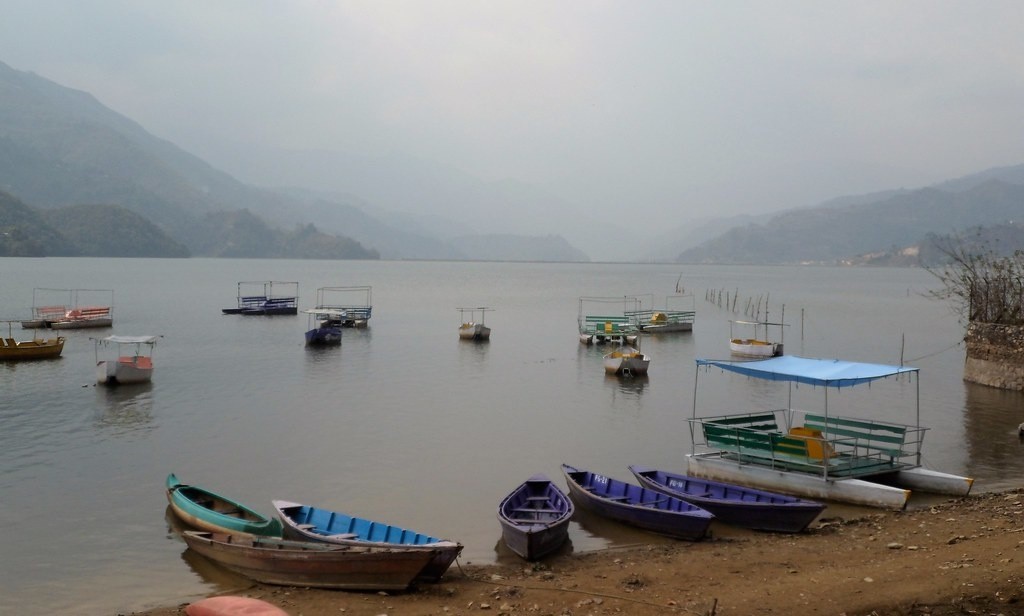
[0,318,66,360]
[221,279,300,316]
[180,530,444,591]
[600,344,652,376]
[684,354,976,513]
[560,462,715,542]
[315,285,373,328]
[165,472,283,539]
[727,319,790,358]
[269,498,467,589]
[576,296,640,347]
[88,334,163,387]
[496,471,576,561]
[458,306,493,342]
[622,293,697,333]
[20,287,115,330]
[627,463,829,534]
[299,309,345,348]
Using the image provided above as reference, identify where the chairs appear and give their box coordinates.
[612,352,623,358]
[612,324,620,333]
[732,340,742,344]
[0,338,4,346]
[630,353,638,357]
[597,324,605,333]
[5,339,17,347]
[463,324,469,329]
[47,339,57,345]
[119,357,131,361]
[137,357,151,367]
[36,338,44,345]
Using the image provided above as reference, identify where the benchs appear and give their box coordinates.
[195,499,212,503]
[690,488,712,497]
[702,413,903,468]
[329,534,358,539]
[582,486,595,489]
[35,306,110,322]
[508,519,555,523]
[297,524,316,529]
[635,499,666,505]
[527,496,550,500]
[510,507,563,513]
[608,496,631,500]
[213,508,244,514]
